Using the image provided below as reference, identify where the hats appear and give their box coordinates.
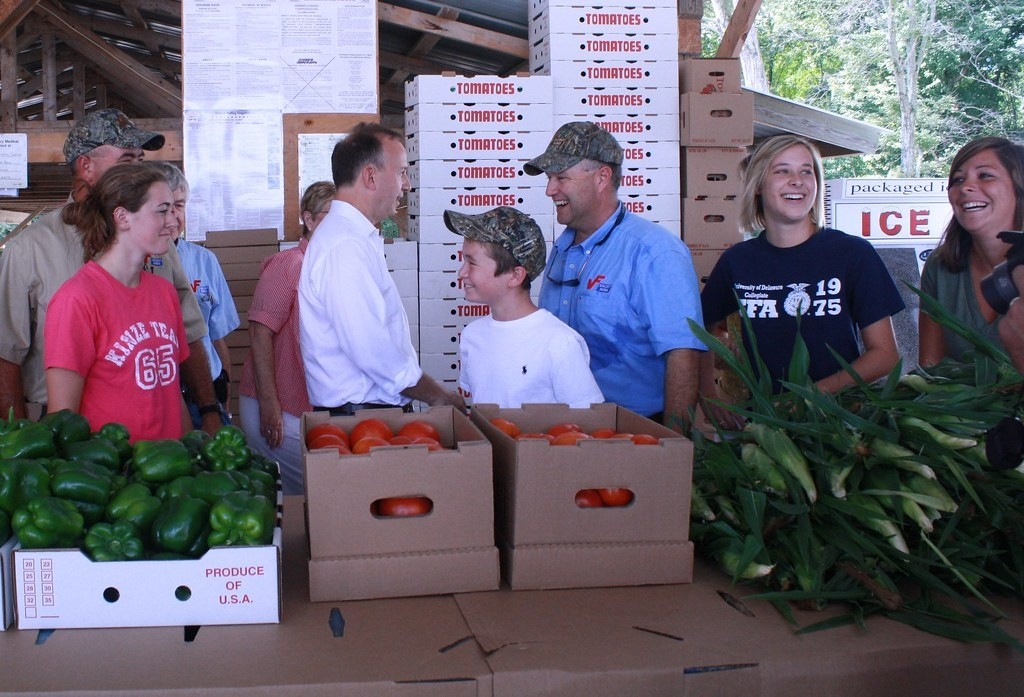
[523,122,622,176]
[444,206,547,281]
[61,107,166,165]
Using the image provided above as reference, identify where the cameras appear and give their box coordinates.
[979,230,1024,315]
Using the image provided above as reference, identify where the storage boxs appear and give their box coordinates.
[297,403,504,604]
[384,1,759,392]
[9,461,284,631]
[470,401,696,592]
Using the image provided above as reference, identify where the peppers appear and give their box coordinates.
[0,406,277,562]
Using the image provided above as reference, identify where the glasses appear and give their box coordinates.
[548,246,595,286]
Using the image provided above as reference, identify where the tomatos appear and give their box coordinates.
[305,417,659,518]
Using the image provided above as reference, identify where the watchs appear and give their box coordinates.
[198,401,224,418]
[226,412,234,421]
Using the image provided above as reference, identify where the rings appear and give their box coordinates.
[719,420,727,428]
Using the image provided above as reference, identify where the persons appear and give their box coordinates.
[297,120,466,416]
[697,134,906,447]
[1,107,224,438]
[241,181,337,496]
[521,119,712,438]
[43,162,193,448]
[141,161,241,431]
[997,264,1024,377]
[918,136,1024,369]
[442,206,608,416]
